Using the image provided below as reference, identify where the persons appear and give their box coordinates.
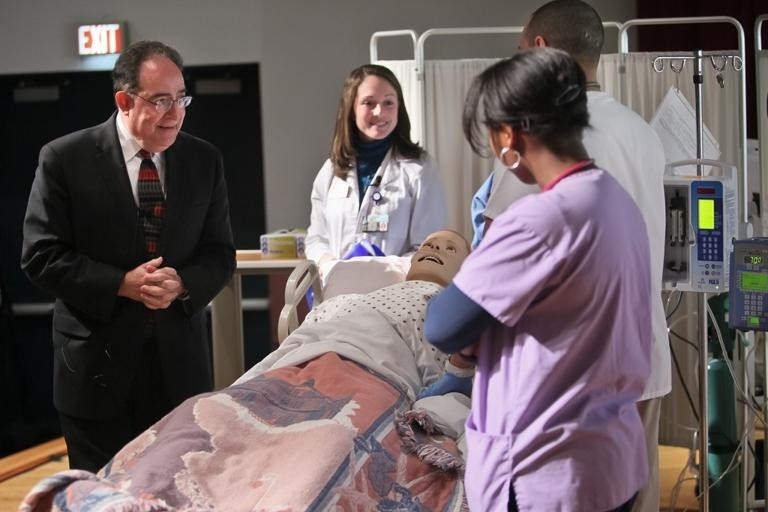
[406,229,472,288]
[303,62,450,292]
[414,0,672,510]
[425,46,653,510]
[20,40,238,473]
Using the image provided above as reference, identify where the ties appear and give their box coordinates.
[135,148,167,259]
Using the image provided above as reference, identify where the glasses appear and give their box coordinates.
[132,92,193,113]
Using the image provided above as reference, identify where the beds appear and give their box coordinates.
[47,255,470,511]
